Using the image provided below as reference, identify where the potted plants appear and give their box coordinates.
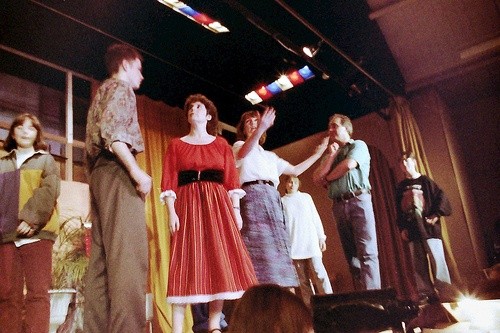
[47,228,93,333]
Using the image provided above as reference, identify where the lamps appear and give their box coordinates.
[303,38,323,58]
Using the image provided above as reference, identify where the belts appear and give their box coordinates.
[333,190,363,201]
[240,179,274,188]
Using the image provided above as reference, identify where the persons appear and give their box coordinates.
[281,174,334,295]
[313,114,381,289]
[84,44,154,333]
[232,106,331,287]
[228,283,312,333]
[394,150,455,303]
[159,95,246,333]
[0,112,60,333]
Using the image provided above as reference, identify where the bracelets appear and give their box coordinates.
[232,207,242,211]
